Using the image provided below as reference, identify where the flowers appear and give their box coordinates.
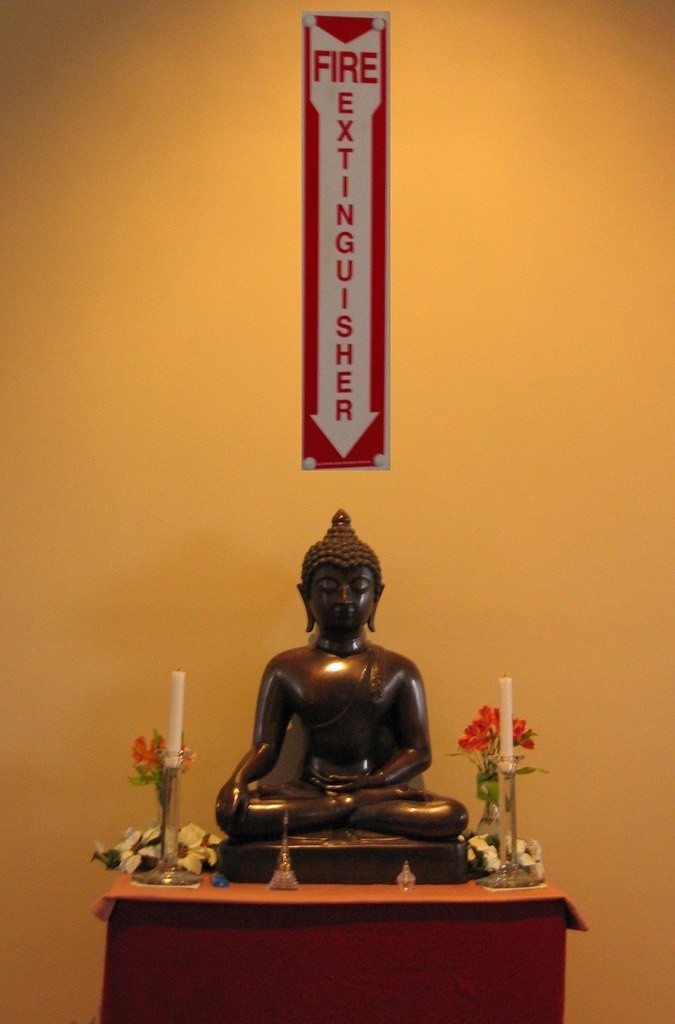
[447,705,550,813]
[91,823,222,876]
[459,834,541,877]
[128,731,194,803]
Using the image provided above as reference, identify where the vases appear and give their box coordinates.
[156,784,171,860]
[472,790,504,833]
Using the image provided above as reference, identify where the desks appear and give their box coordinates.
[91,869,590,1024]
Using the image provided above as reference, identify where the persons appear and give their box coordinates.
[214,508,472,842]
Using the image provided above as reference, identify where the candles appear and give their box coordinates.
[164,671,186,765]
[499,675,514,771]
